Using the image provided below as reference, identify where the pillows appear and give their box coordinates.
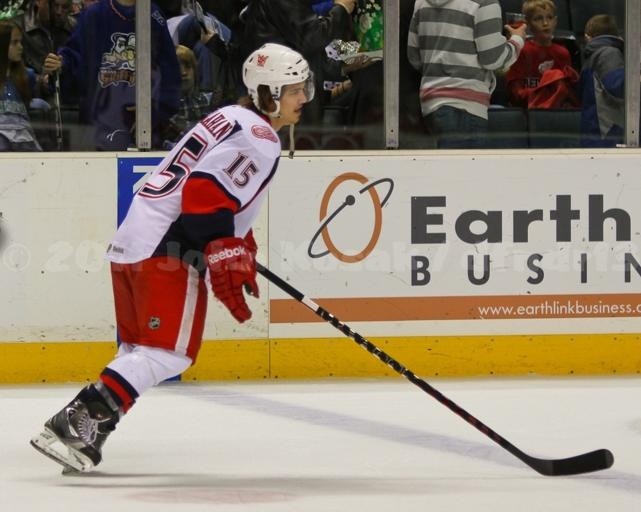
[241,41,316,119]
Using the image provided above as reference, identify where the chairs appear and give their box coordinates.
[43,382,117,467]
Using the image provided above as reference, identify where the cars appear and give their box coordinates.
[504,12,527,39]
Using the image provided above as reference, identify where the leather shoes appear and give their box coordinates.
[205,229,260,324]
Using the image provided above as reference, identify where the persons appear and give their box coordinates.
[1,1,384,153]
[45,41,310,465]
[582,13,624,148]
[504,3,580,108]
[407,0,527,150]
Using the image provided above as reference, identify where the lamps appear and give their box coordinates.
[485,108,583,148]
